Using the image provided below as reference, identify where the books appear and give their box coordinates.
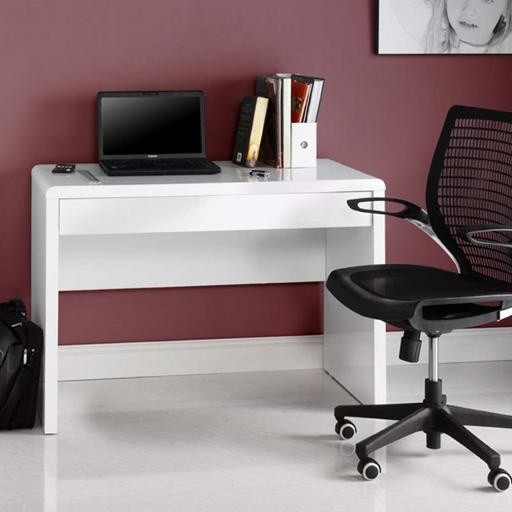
[253,72,325,169]
[230,94,270,170]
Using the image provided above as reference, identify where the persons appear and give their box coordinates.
[418,1,511,55]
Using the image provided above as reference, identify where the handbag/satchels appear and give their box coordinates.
[0,299,43,430]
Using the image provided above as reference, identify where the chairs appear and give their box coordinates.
[325,105,512,494]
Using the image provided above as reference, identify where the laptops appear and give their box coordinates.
[98,89,222,175]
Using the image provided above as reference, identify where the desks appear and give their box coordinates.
[31,158,387,436]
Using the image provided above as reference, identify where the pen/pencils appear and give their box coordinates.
[249,170,270,177]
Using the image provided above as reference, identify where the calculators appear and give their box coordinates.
[52,164,75,173]
